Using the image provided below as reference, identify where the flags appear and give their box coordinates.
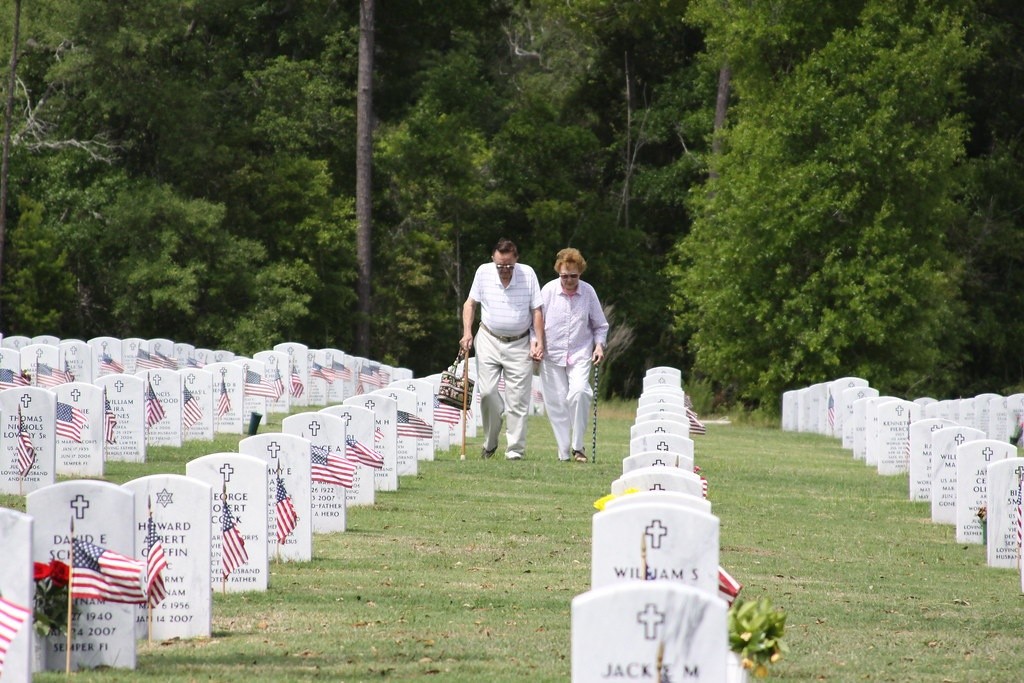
[0,348,232,481]
[220,499,249,581]
[397,410,433,438]
[72,517,168,609]
[303,439,384,489]
[276,477,297,545]
[433,375,544,430]
[244,359,390,403]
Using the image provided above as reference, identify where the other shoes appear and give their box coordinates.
[572,449,588,463]
[481,446,498,459]
[508,456,521,461]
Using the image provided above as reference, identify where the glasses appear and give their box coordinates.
[558,272,580,279]
[493,257,515,270]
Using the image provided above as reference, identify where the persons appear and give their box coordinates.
[459,240,545,461]
[529,247,609,463]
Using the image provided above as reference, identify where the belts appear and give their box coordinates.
[479,321,530,342]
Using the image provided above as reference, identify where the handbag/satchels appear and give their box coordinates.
[438,347,475,411]
